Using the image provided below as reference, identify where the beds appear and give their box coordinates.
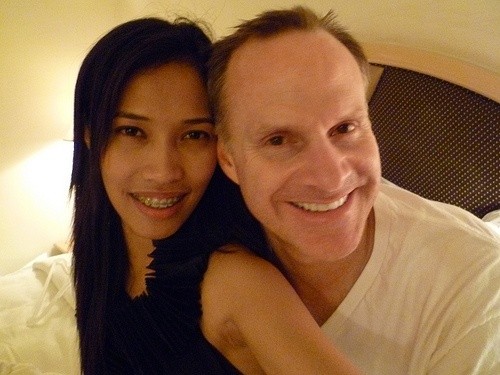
[0,44,499,373]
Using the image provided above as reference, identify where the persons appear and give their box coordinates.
[70,17,363,375]
[210,6,500,375]
